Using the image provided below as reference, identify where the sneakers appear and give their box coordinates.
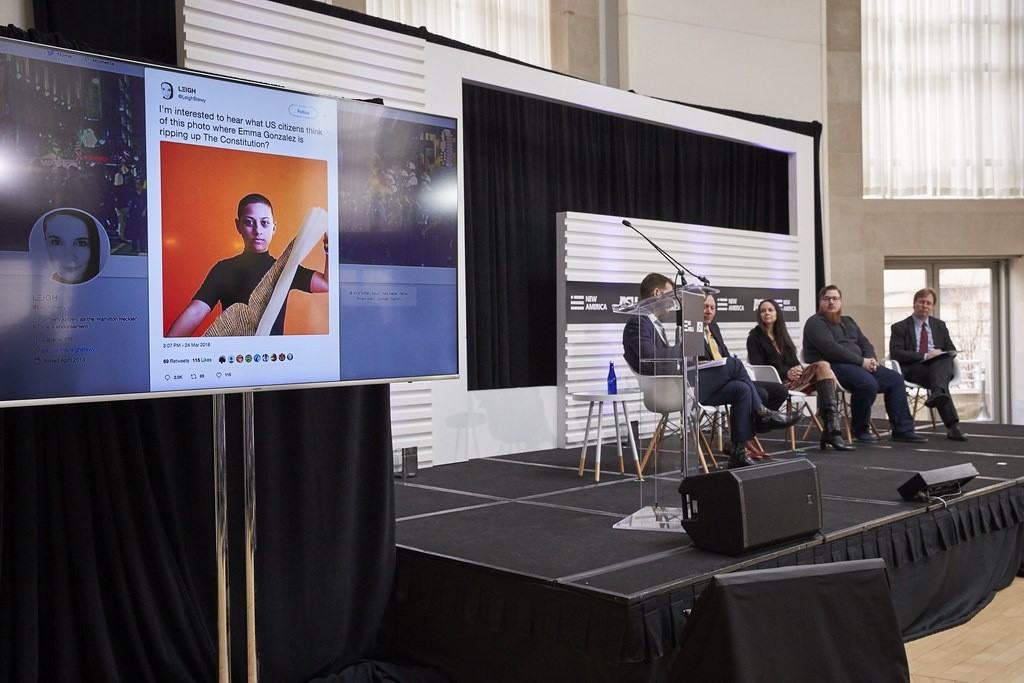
[853,431,877,443]
[892,431,928,441]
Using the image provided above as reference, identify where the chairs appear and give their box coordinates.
[623,354,963,470]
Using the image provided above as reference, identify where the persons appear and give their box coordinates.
[43,209,101,285]
[166,193,328,338]
[161,82,173,99]
[622,272,805,470]
[697,295,787,461]
[25,156,147,255]
[889,287,968,441]
[746,300,855,451]
[802,285,928,442]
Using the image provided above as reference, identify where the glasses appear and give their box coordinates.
[820,296,841,302]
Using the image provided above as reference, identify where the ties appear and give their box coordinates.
[918,322,928,353]
[703,327,723,361]
[655,319,669,347]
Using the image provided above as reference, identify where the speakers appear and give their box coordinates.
[679,458,823,557]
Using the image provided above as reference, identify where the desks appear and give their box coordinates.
[573,390,645,481]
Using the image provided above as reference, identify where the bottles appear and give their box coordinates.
[607,360,617,395]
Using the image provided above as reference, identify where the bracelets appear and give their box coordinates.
[323,243,328,254]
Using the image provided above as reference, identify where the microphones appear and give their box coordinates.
[622,220,710,287]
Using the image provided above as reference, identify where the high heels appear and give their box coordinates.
[821,405,835,425]
[820,429,856,450]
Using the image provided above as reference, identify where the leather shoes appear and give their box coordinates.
[744,446,763,460]
[727,454,758,468]
[925,392,949,408]
[946,428,968,441]
[746,440,771,458]
[755,410,802,433]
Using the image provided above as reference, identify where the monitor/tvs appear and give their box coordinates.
[0,36,459,409]
